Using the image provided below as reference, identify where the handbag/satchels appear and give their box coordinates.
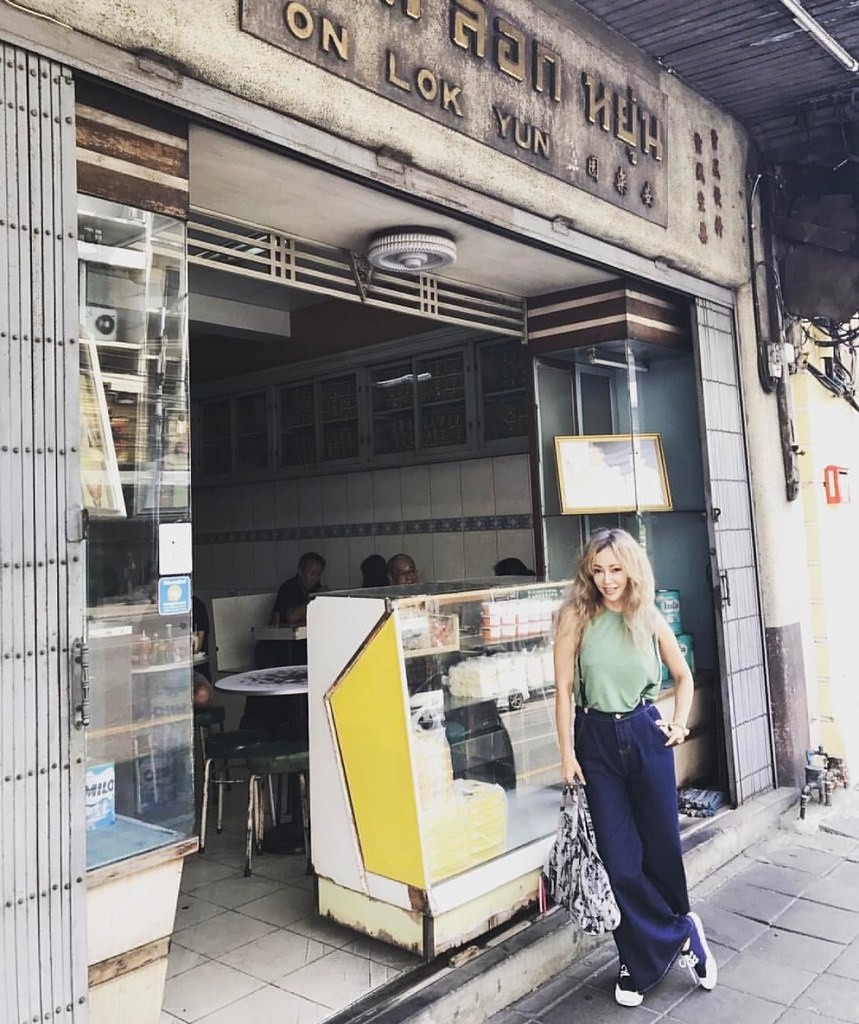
[540,777,621,935]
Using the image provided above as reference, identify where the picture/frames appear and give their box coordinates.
[551,432,674,515]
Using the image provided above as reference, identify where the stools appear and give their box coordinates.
[198,704,310,876]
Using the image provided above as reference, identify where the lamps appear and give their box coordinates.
[367,236,457,274]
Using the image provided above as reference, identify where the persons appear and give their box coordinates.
[493,558,537,577]
[267,550,422,661]
[553,527,718,1009]
[135,571,215,706]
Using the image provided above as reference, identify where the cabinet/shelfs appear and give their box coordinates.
[87,462,187,1024]
[404,614,557,746]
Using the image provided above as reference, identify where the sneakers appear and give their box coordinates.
[614,959,644,1006]
[679,912,717,989]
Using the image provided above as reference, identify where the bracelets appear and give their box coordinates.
[669,720,690,737]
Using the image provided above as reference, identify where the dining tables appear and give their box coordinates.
[214,665,309,737]
[132,653,210,677]
[253,621,307,641]
